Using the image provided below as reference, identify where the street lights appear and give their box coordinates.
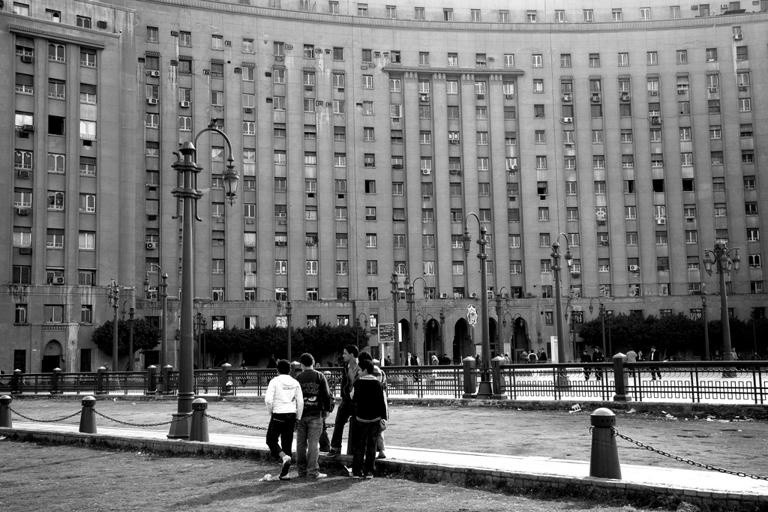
[549,231,574,393]
[388,259,411,378]
[167,125,242,440]
[589,295,607,362]
[702,234,741,378]
[354,309,370,365]
[495,285,511,357]
[106,259,170,397]
[405,276,430,352]
[462,210,495,399]
[564,294,577,363]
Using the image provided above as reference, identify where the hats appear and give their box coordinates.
[299,353,314,367]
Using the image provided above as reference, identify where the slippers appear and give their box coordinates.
[376,455,385,459]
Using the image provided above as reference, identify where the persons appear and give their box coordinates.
[239,359,249,386]
[291,352,332,483]
[355,351,386,460]
[348,359,389,480]
[371,357,386,384]
[263,358,305,481]
[386,346,763,384]
[326,343,363,457]
[289,360,331,454]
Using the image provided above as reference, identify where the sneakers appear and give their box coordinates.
[327,448,341,458]
[365,472,373,479]
[353,473,362,479]
[315,473,327,479]
[280,455,291,476]
